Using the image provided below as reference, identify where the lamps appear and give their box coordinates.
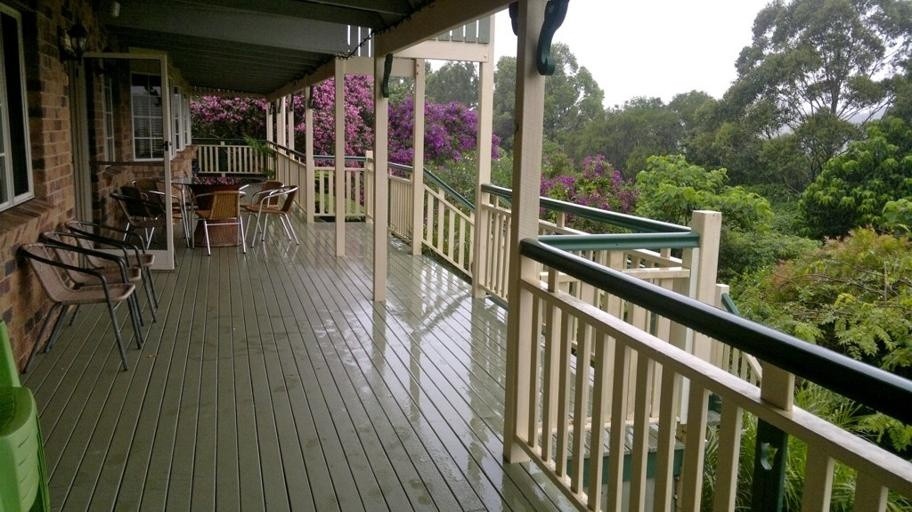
[62,16,89,63]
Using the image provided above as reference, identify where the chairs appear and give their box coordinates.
[16,219,160,372]
[109,174,304,257]
